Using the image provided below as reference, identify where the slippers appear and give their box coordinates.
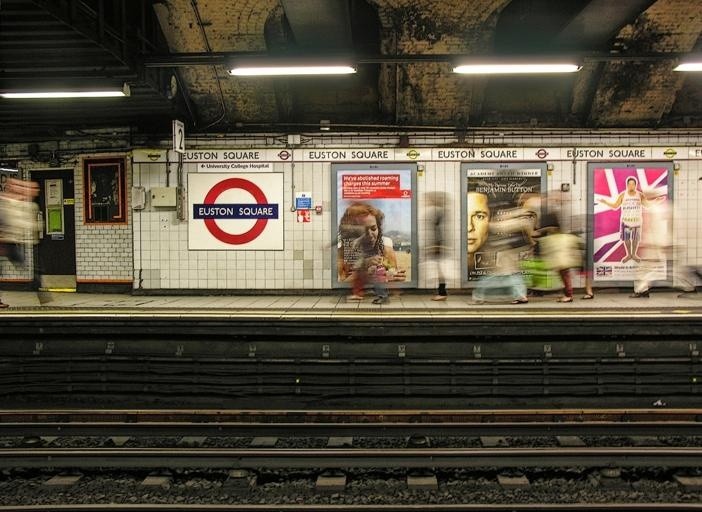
[512,299,529,304]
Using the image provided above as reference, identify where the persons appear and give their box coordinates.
[371,279,390,305]
[628,197,668,299]
[1,177,59,311]
[565,207,595,299]
[418,191,455,301]
[541,192,574,301]
[597,174,666,264]
[468,259,528,305]
[336,199,407,281]
[467,181,498,278]
[346,257,366,301]
[490,192,541,248]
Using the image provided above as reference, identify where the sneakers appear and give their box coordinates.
[631,290,650,298]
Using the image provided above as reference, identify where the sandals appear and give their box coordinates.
[557,296,574,303]
[581,293,595,300]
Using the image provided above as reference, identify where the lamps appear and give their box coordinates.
[1,84,130,101]
[673,60,701,70]
[454,62,580,75]
[227,64,355,75]
